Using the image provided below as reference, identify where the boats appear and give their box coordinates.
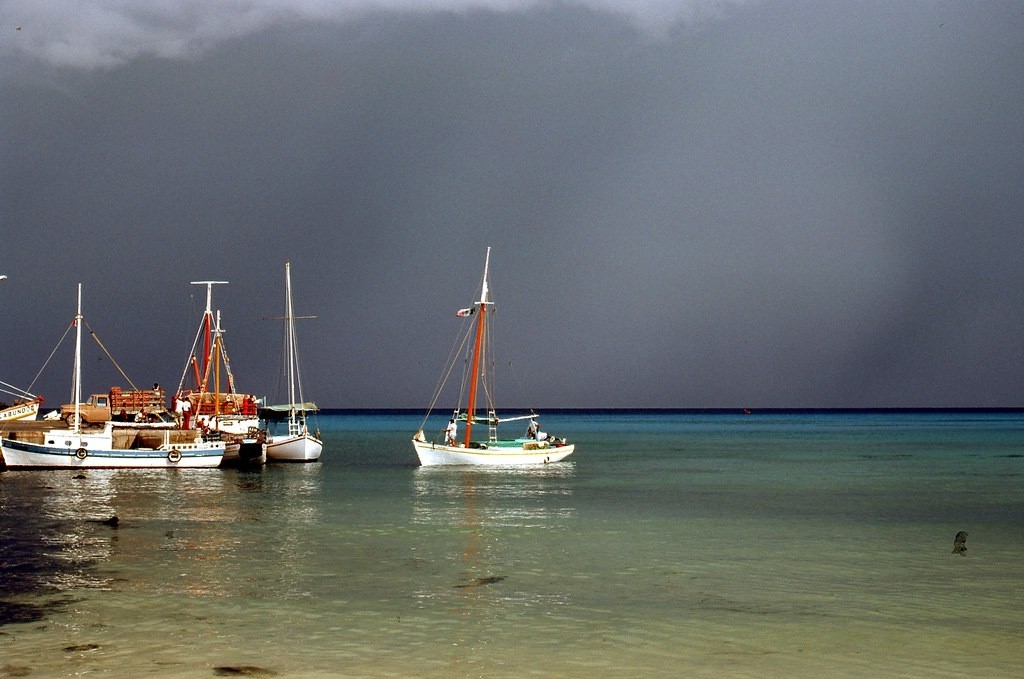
[0,380,44,420]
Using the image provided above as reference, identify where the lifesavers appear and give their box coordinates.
[167,450,182,463]
[75,448,88,460]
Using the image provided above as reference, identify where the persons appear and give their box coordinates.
[134,408,146,423]
[173,397,184,430]
[204,424,211,442]
[197,418,206,430]
[152,383,161,408]
[182,397,193,430]
[120,403,129,422]
[443,418,457,446]
[300,420,308,437]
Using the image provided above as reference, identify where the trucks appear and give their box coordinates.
[60,387,168,428]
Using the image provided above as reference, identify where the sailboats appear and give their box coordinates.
[0,284,226,468]
[267,261,323,462]
[172,281,266,459]
[413,246,575,468]
